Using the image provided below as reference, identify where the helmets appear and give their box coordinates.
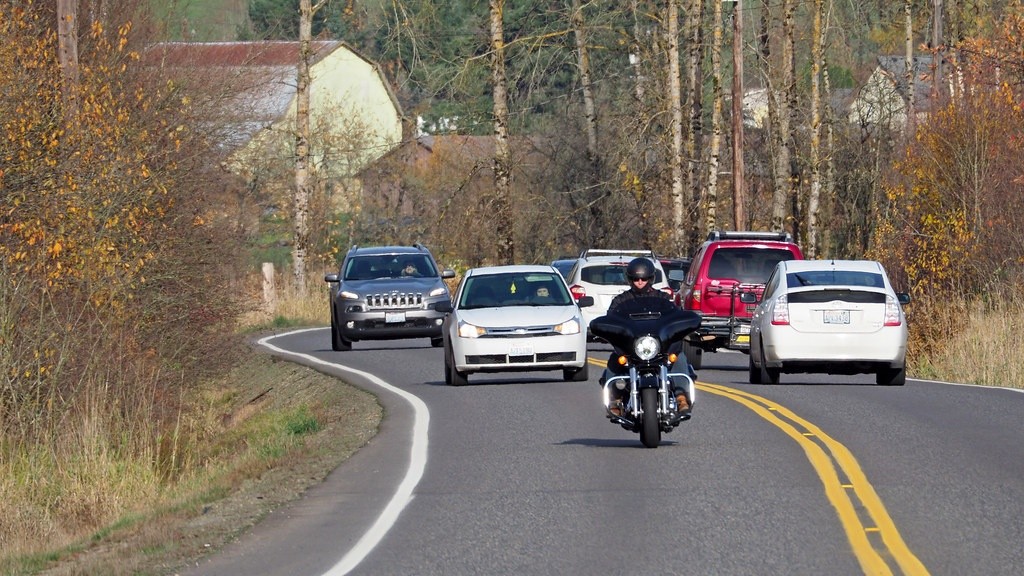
[627,258,656,294]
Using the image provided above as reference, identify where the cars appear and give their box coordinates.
[442,265,594,387]
[747,257,908,386]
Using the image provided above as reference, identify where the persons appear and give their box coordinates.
[536,285,549,297]
[404,260,417,276]
[604,257,692,422]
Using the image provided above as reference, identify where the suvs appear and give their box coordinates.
[569,248,673,343]
[324,242,451,351]
[679,230,807,372]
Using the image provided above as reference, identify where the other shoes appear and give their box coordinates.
[676,395,689,412]
[606,407,621,417]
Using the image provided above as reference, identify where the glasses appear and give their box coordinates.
[631,277,650,282]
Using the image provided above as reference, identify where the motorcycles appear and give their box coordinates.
[588,306,705,447]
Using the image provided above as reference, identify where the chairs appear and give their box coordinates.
[763,259,782,281]
[350,260,378,280]
[603,268,624,283]
[470,286,500,306]
[710,254,738,276]
